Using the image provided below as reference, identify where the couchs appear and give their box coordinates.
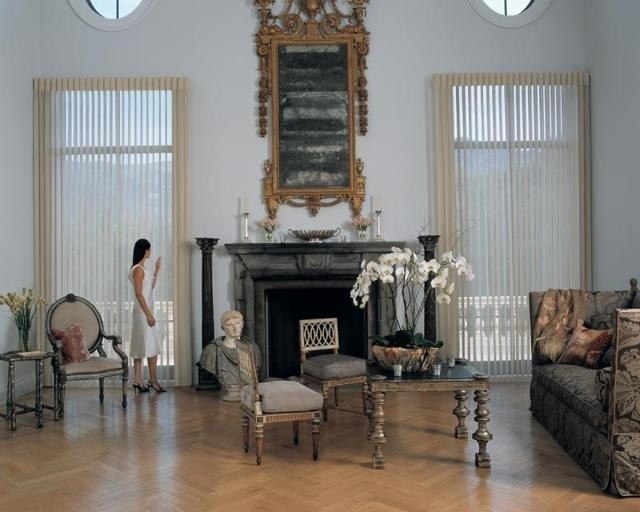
[528,278,640,498]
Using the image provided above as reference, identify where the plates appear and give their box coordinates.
[288,228,341,242]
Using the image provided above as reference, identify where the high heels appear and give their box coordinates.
[147,380,167,393]
[133,381,149,396]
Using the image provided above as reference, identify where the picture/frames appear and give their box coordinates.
[251,0,371,219]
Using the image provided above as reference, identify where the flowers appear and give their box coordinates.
[350,246,476,348]
[352,213,378,231]
[0,286,48,349]
[258,217,283,234]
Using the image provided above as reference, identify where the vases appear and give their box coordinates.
[265,232,274,243]
[371,335,443,373]
[357,229,369,242]
[16,329,32,352]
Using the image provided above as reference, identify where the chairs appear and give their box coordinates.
[236,340,323,466]
[46,292,128,417]
[298,317,366,423]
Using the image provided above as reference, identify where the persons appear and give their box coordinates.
[127,238,167,393]
[199,310,263,389]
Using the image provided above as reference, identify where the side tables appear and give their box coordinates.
[0,351,56,431]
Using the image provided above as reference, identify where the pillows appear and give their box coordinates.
[50,321,91,365]
[556,320,612,369]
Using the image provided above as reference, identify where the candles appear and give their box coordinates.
[377,195,382,210]
[244,198,249,212]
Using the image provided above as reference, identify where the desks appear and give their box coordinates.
[366,358,493,469]
